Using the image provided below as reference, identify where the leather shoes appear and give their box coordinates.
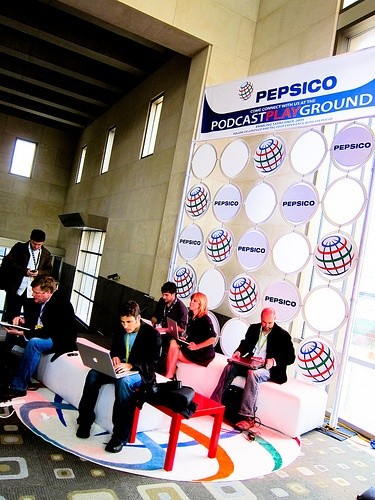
[0,388,27,401]
[76,424,92,439]
[104,437,127,453]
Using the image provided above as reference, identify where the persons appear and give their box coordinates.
[76,301,162,453]
[0,274,78,396]
[165,292,217,381]
[151,281,187,364]
[2,229,53,328]
[210,307,295,431]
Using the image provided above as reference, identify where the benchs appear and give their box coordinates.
[36,351,168,434]
[175,351,329,438]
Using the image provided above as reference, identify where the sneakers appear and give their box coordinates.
[234,419,255,431]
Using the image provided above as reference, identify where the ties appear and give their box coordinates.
[125,334,129,364]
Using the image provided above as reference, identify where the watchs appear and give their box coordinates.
[16,331,20,337]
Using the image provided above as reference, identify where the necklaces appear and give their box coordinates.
[126,334,130,363]
[258,330,266,354]
[30,245,41,270]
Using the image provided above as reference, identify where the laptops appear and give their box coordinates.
[227,356,265,370]
[76,337,139,379]
[167,317,190,344]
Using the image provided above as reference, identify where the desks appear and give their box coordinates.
[129,392,225,472]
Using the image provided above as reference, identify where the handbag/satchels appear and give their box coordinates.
[220,385,244,424]
[0,242,20,290]
[135,373,195,412]
